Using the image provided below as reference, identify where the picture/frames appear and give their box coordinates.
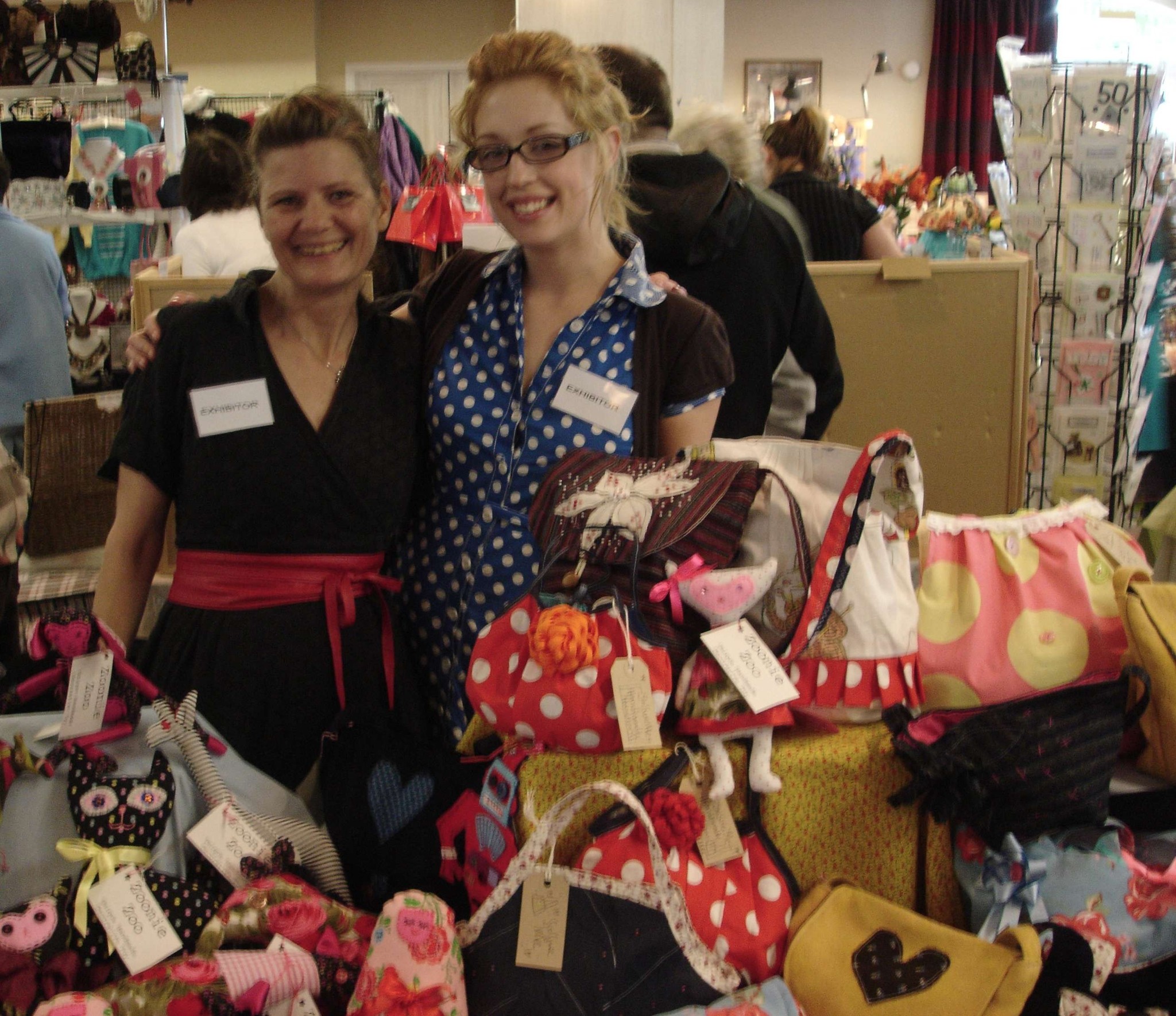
[744,59,822,131]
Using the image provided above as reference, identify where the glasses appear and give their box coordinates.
[461,128,592,176]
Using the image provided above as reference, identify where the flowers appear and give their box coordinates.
[837,130,996,232]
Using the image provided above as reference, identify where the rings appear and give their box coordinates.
[671,284,681,294]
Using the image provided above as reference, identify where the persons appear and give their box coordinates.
[595,46,844,440]
[0,150,73,474]
[125,30,734,791]
[92,92,690,916]
[173,134,279,278]
[762,106,912,260]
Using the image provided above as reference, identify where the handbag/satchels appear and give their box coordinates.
[385,153,494,253]
[464,433,1176,1016]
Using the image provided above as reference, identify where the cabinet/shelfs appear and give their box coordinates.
[1,78,162,395]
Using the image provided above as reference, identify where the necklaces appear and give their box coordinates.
[263,284,359,383]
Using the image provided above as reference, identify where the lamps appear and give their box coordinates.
[903,60,919,82]
[862,51,893,118]
[766,73,798,122]
